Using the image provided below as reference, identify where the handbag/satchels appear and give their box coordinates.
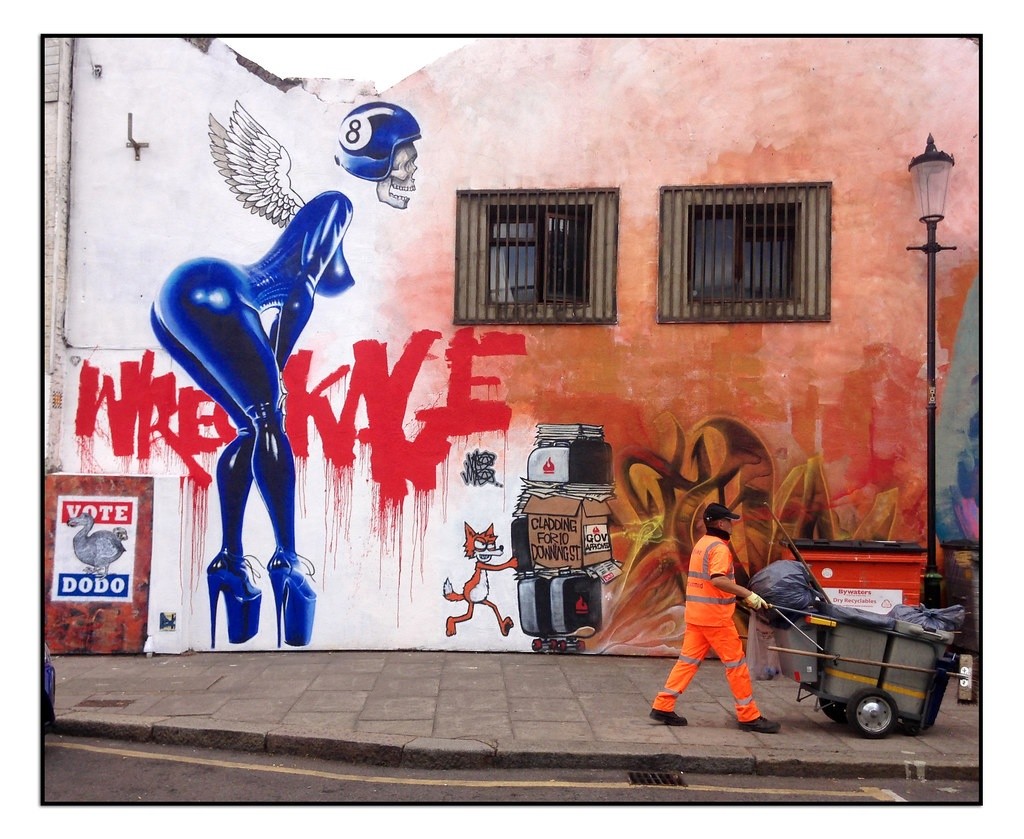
[747,610,782,680]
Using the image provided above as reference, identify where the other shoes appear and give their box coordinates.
[649,708,688,726]
[739,716,780,734]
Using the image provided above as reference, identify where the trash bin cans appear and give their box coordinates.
[778,536,928,616]
[768,605,818,684]
[942,539,981,654]
[800,599,896,705]
[878,619,955,716]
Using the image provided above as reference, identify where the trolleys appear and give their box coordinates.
[738,597,950,739]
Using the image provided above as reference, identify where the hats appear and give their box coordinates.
[703,503,741,520]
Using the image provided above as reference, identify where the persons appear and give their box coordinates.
[649,503,781,734]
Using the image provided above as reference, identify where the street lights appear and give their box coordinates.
[903,131,960,621]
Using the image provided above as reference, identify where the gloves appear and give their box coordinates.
[744,591,768,610]
